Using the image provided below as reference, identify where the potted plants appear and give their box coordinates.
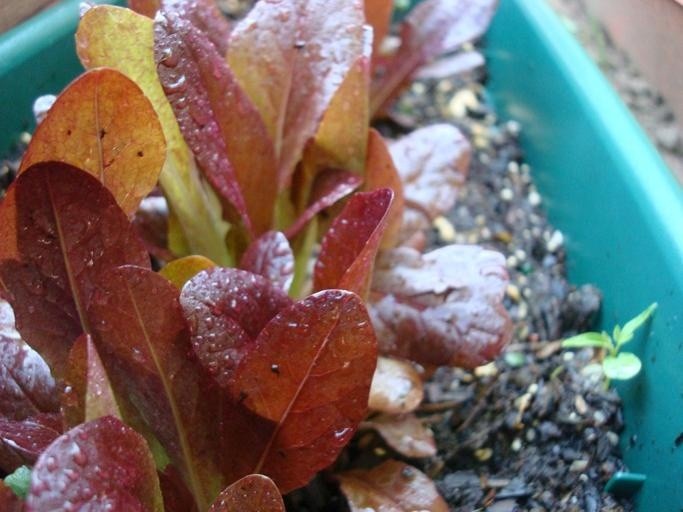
[1,0,681,510]
